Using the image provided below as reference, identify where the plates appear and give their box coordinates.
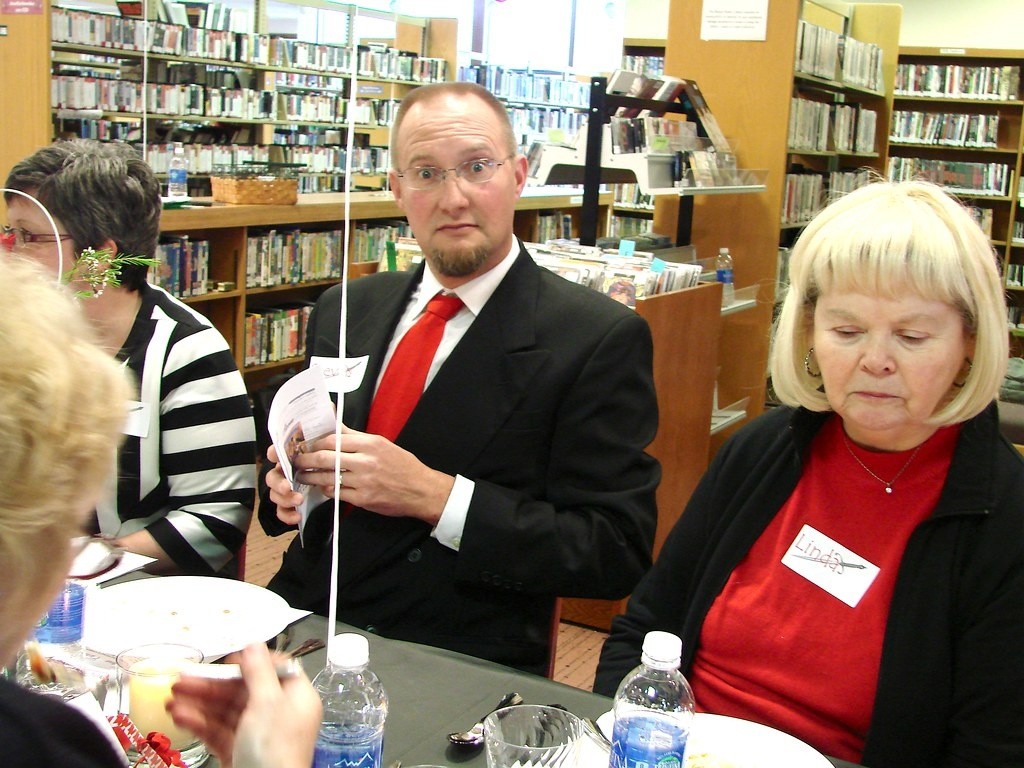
[577,713,835,768]
[83,575,291,660]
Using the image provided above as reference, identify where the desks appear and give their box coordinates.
[98,569,868,768]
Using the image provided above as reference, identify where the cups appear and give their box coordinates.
[117,642,221,768]
[482,705,581,768]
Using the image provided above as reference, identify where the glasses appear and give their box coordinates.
[2,225,73,245]
[396,154,514,191]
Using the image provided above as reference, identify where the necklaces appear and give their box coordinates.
[843,435,923,493]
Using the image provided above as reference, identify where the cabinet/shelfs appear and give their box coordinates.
[0,0,1024,631]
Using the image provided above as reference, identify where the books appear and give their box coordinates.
[887,65,1024,333]
[51,0,424,367]
[774,19,882,299]
[458,64,590,187]
[606,55,737,237]
[523,215,703,308]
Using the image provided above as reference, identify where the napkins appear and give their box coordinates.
[68,533,157,587]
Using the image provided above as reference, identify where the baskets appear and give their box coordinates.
[210,164,299,205]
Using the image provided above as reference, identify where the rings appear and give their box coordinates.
[340,473,343,484]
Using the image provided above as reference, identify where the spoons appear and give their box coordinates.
[447,692,523,746]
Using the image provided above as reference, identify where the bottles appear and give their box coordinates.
[167,147,188,198]
[308,632,389,768]
[716,247,734,307]
[607,631,693,767]
[14,576,88,705]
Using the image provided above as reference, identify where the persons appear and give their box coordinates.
[0,254,323,768]
[592,178,1024,768]
[3,138,256,580]
[258,84,663,678]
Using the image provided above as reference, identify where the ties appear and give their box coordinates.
[365,294,465,443]
